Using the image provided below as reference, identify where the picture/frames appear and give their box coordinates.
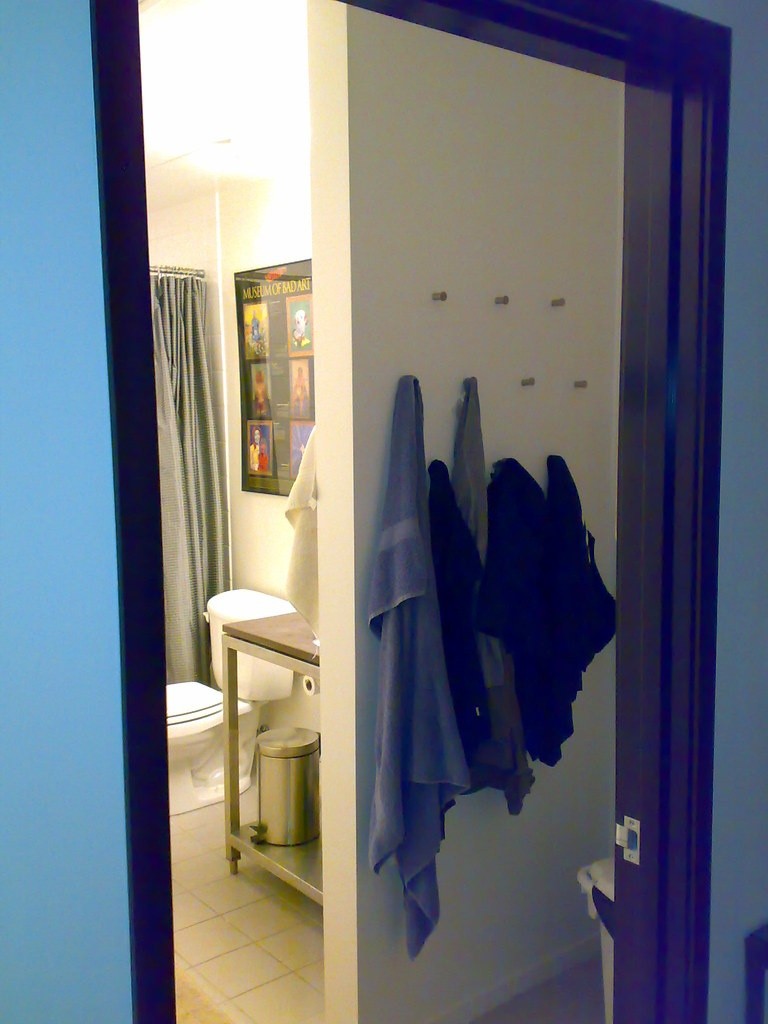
[241,294,316,483]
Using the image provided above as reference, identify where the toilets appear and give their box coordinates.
[165,589,299,816]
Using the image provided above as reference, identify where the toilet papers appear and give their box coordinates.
[302,675,319,696]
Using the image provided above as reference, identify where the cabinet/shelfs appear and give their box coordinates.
[221,611,323,907]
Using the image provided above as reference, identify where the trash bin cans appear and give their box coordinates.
[249,726,321,846]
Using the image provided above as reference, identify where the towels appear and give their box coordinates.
[283,424,320,644]
[369,371,619,965]
